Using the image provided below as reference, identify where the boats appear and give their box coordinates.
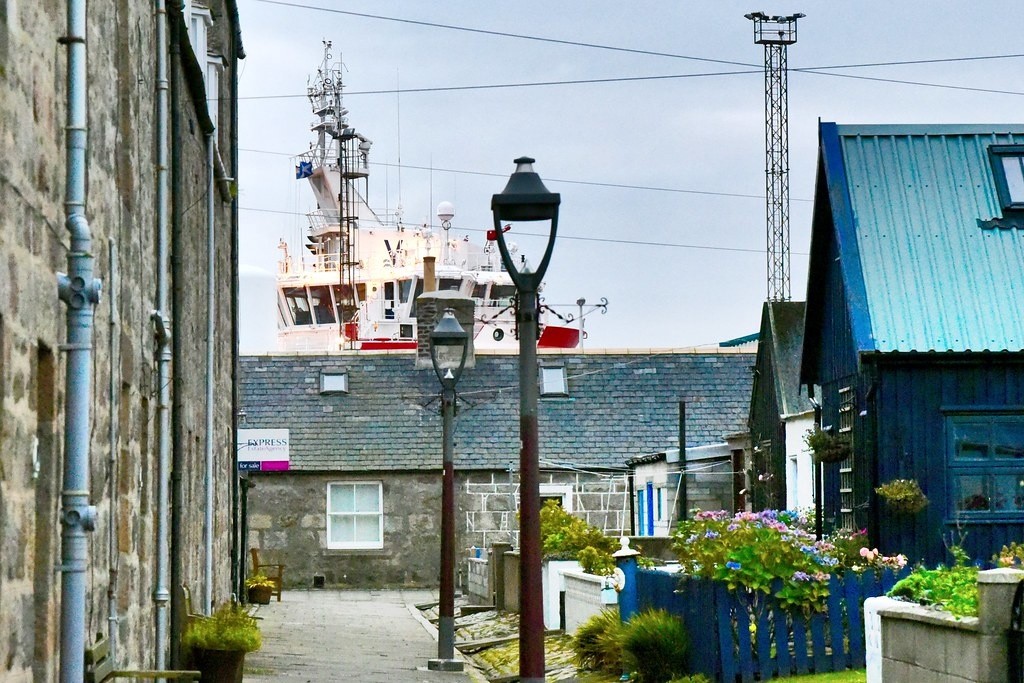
[266,37,586,350]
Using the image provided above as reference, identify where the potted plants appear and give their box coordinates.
[801,426,852,464]
[245,576,278,605]
[875,479,931,514]
[516,498,688,683]
[180,599,263,683]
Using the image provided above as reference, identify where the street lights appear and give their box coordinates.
[426,303,470,673]
[489,149,563,682]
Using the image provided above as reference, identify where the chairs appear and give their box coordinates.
[181,582,247,638]
[249,548,286,601]
[85,632,201,683]
